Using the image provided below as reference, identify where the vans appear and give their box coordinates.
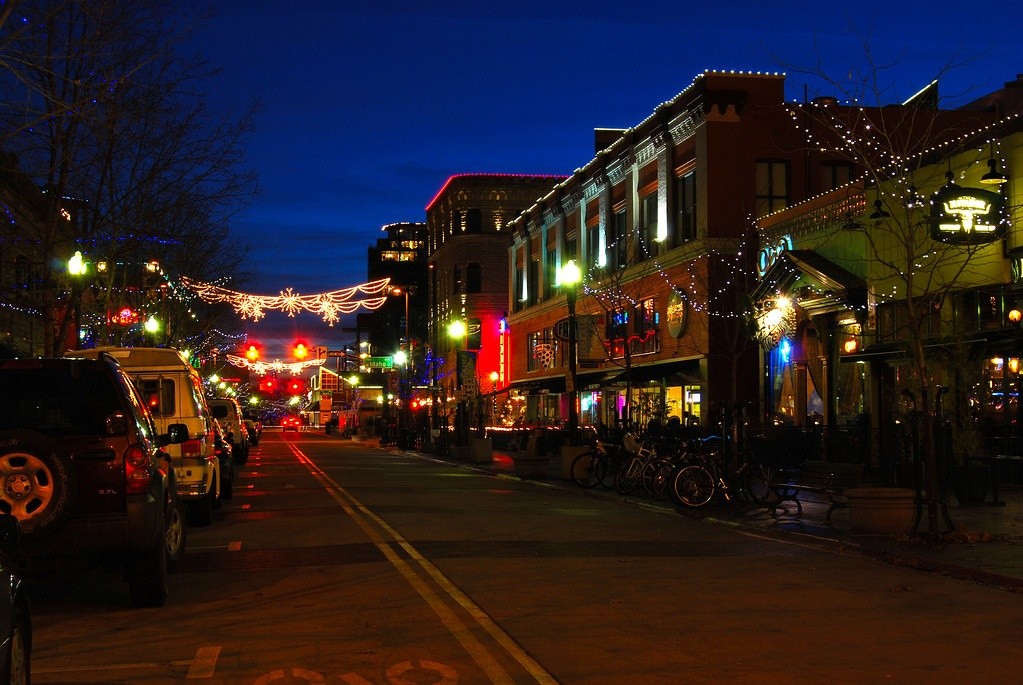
[205,398,250,464]
[66,347,223,528]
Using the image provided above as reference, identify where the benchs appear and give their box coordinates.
[768,459,866,528]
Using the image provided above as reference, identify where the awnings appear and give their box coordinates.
[601,362,703,388]
[508,371,606,397]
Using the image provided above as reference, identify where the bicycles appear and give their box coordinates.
[568,427,783,509]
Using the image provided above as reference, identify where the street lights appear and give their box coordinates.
[559,259,583,429]
[67,250,88,350]
[391,352,407,398]
[145,315,160,346]
[447,320,467,415]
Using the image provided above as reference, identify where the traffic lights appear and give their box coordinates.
[248,346,257,364]
[296,344,305,361]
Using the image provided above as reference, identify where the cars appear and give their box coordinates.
[208,416,236,503]
[283,418,300,432]
[242,419,261,447]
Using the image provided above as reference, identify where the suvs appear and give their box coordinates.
[0,352,192,613]
